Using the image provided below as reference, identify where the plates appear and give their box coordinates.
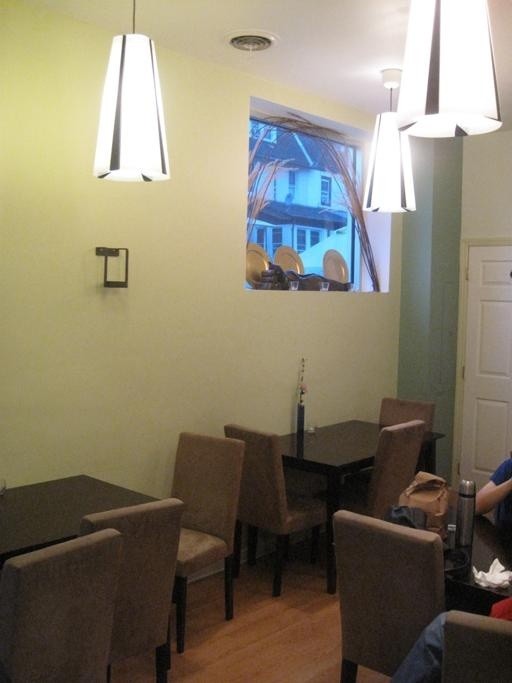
[246,242,271,287]
[273,244,306,275]
[322,249,351,284]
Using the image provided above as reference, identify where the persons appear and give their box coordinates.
[473,454,510,527]
[387,594,512,682]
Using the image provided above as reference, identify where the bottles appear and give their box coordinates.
[455,478,474,545]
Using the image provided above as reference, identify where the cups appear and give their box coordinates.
[287,279,299,290]
[317,281,330,290]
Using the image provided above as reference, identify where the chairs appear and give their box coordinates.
[354,419,426,522]
[223,424,326,598]
[170,432,246,654]
[80,498,184,683]
[441,611,512,683]
[378,397,434,432]
[0,528,125,683]
[332,510,447,683]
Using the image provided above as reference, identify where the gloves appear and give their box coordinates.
[474,558,509,590]
[472,565,511,584]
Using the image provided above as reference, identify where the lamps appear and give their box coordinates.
[94,0,170,182]
[397,0,502,138]
[361,68,416,214]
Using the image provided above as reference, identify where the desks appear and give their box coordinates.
[1,475,161,554]
[446,500,512,597]
[271,421,446,597]
